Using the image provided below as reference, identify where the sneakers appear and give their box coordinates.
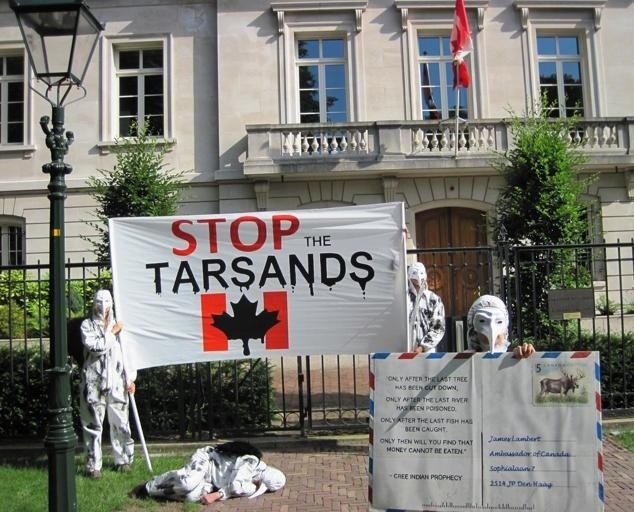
[84,464,130,479]
[130,483,147,499]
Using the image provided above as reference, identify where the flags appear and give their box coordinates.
[450,0,473,90]
[422,50,441,119]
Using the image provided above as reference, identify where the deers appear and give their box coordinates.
[540,368,585,400]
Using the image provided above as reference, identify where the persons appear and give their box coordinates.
[128,441,287,506]
[78,289,136,480]
[407,262,446,353]
[466,294,535,359]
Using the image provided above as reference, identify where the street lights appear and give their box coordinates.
[4,0,108,511]
